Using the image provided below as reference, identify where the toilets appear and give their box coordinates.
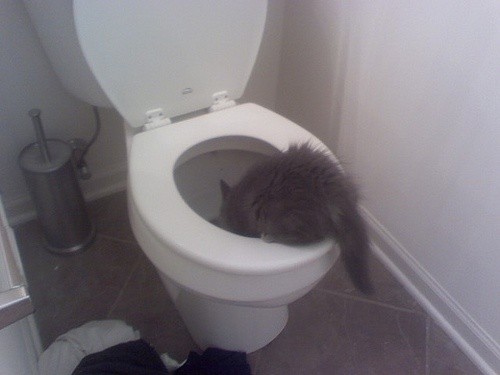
[23,0,347,355]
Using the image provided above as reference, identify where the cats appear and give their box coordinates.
[220,136,377,298]
